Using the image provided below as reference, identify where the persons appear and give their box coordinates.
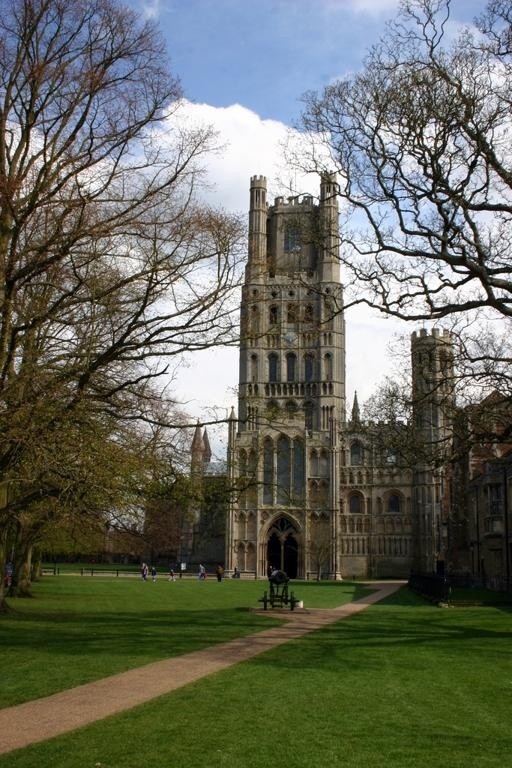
[234,566,237,574]
[216,564,224,582]
[198,564,207,579]
[168,568,175,582]
[268,565,276,581]
[151,566,157,582]
[141,565,148,581]
